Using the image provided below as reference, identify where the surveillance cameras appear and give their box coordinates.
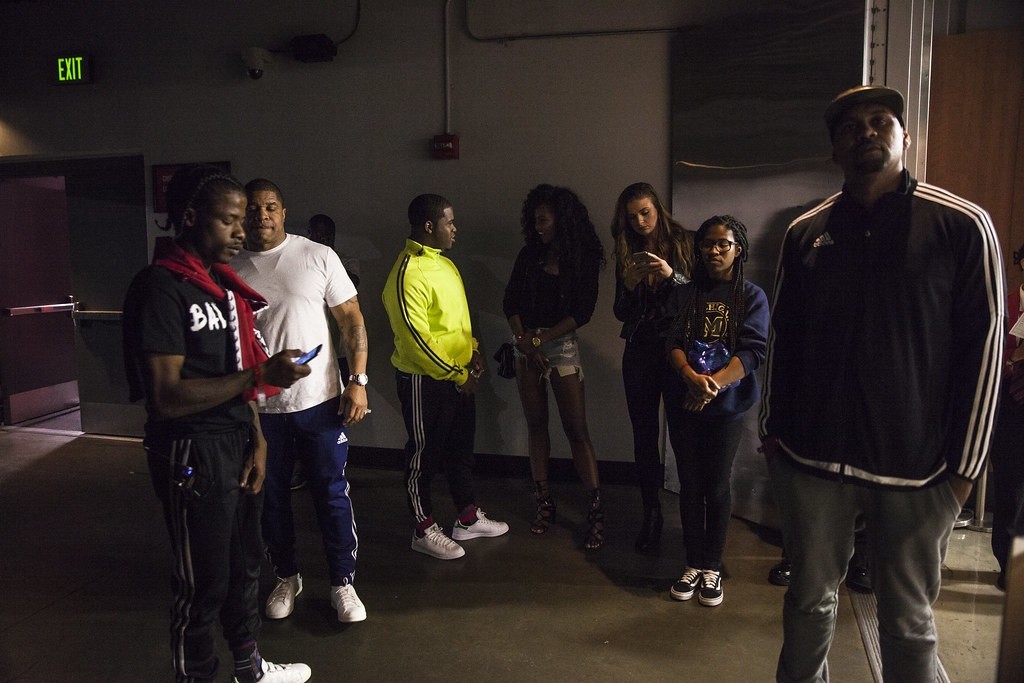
[240,46,271,80]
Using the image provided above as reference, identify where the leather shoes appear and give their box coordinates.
[768,561,791,586]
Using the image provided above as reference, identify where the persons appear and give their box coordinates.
[766,511,873,591]
[502,183,608,551]
[662,216,770,607]
[757,84,1009,683]
[382,193,509,560]
[610,182,702,557]
[288,215,360,490]
[121,165,311,683]
[228,178,369,622]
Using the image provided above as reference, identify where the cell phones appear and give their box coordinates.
[293,344,323,365]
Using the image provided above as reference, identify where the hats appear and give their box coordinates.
[825,85,905,129]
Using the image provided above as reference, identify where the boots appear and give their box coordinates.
[635,493,664,555]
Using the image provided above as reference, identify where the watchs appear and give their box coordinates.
[349,373,368,385]
[532,334,542,347]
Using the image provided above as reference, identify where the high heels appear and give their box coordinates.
[530,479,557,534]
[583,488,606,553]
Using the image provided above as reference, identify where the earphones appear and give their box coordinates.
[415,248,425,256]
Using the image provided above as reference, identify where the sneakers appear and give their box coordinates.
[670,566,702,600]
[698,568,723,607]
[411,522,465,560]
[231,658,311,683]
[452,508,509,540]
[331,584,367,622]
[265,572,303,619]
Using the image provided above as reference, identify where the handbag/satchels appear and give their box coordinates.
[493,342,515,379]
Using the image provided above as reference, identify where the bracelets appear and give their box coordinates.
[516,334,526,343]
[678,363,688,379]
[251,364,264,389]
